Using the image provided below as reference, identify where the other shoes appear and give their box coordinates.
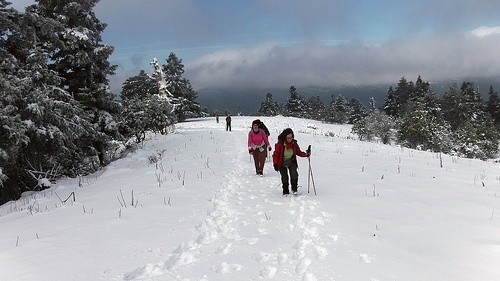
[259,168,264,177]
[292,191,298,195]
[282,193,289,197]
[256,170,259,176]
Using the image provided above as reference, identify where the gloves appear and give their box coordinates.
[249,150,254,154]
[269,147,272,151]
[274,166,279,172]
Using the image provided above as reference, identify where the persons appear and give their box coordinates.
[248,120,272,177]
[226,114,232,131]
[256,119,270,140]
[272,128,311,197]
